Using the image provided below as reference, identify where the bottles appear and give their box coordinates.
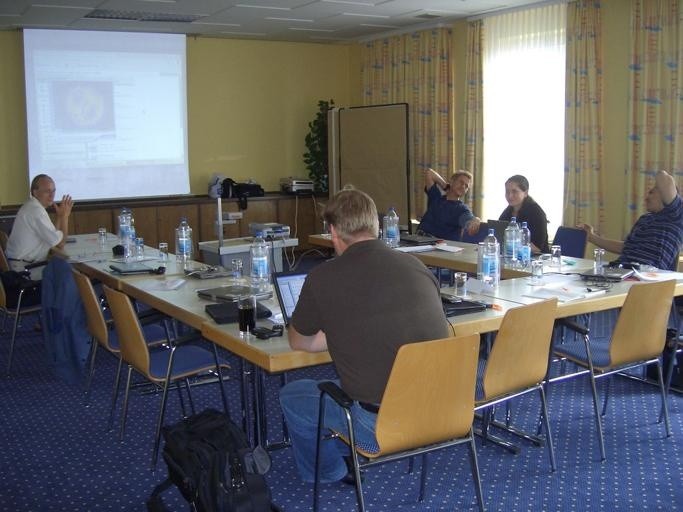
[479,214,532,292]
[251,233,270,291]
[118,205,135,252]
[382,207,400,246]
[175,218,194,269]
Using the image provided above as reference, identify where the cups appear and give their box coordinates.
[98,227,107,249]
[551,245,563,273]
[229,259,257,334]
[454,271,468,297]
[123,238,170,266]
[532,261,545,285]
[593,248,606,274]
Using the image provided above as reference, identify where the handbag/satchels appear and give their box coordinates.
[143,406,275,512]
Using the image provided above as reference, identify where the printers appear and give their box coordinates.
[280,177,314,193]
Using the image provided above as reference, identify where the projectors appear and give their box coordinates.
[250,222,291,241]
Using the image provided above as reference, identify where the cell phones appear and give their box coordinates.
[272,324,283,332]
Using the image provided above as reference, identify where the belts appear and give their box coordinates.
[8,258,35,263]
[355,400,384,416]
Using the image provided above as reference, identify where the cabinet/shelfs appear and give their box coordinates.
[112,197,203,264]
[200,196,279,242]
[278,193,329,260]
[49,199,113,235]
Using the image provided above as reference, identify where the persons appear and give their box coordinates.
[4,174,74,331]
[499,175,551,254]
[278,190,447,486]
[575,169,683,271]
[414,168,480,242]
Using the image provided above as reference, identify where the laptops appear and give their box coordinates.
[487,221,510,247]
[399,234,443,245]
[271,269,311,327]
[109,262,154,275]
[197,285,272,304]
[581,266,634,282]
